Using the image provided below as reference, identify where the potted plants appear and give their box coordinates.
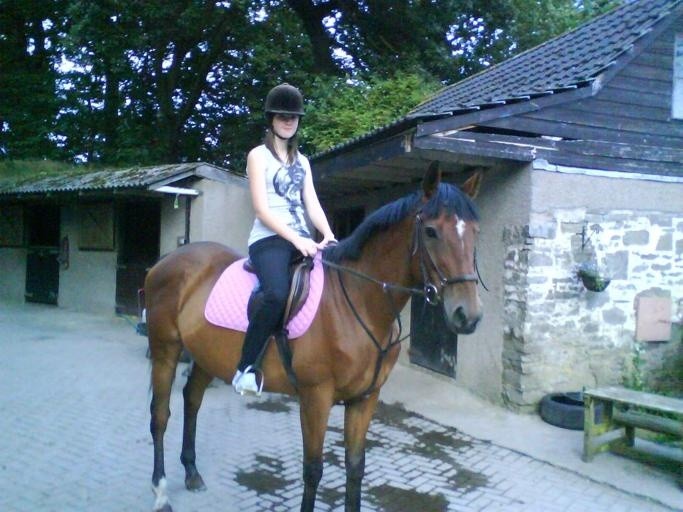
[569,261,614,293]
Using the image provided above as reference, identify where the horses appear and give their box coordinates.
[144,160,483,512]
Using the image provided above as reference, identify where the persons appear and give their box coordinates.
[231,82,338,397]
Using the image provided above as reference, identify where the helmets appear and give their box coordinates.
[265,82,306,116]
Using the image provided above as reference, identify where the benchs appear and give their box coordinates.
[581,383,683,478]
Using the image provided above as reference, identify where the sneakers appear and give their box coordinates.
[231,370,258,393]
